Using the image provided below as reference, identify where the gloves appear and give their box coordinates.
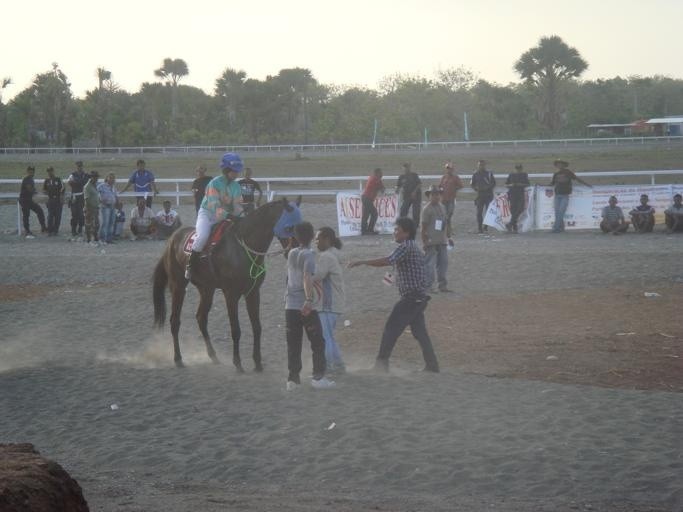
[226,214,242,223]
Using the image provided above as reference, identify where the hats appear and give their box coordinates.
[87,171,98,177]
[47,167,54,172]
[425,184,444,198]
[555,158,569,168]
[76,160,83,168]
[445,163,452,168]
[27,166,35,170]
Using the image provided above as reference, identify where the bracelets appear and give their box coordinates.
[305,296,313,303]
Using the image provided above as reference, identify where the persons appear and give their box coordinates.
[629,194,655,233]
[664,194,683,234]
[472,160,496,234]
[396,162,421,227]
[505,163,529,233]
[360,168,385,234]
[310,226,348,378]
[439,162,460,233]
[282,220,336,391]
[547,160,583,233]
[419,183,458,294]
[17,157,263,245]
[601,196,628,234]
[184,153,247,281]
[344,216,440,376]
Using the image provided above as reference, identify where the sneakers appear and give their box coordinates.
[414,367,439,374]
[506,222,518,232]
[286,381,301,390]
[426,284,452,295]
[130,235,154,241]
[356,363,388,374]
[478,229,488,234]
[312,378,334,388]
[71,236,118,247]
[42,227,58,235]
[361,228,379,235]
[27,234,36,239]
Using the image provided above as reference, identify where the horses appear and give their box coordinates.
[152,195,302,374]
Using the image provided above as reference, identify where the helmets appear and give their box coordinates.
[222,153,242,171]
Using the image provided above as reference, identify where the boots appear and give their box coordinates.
[184,249,201,279]
